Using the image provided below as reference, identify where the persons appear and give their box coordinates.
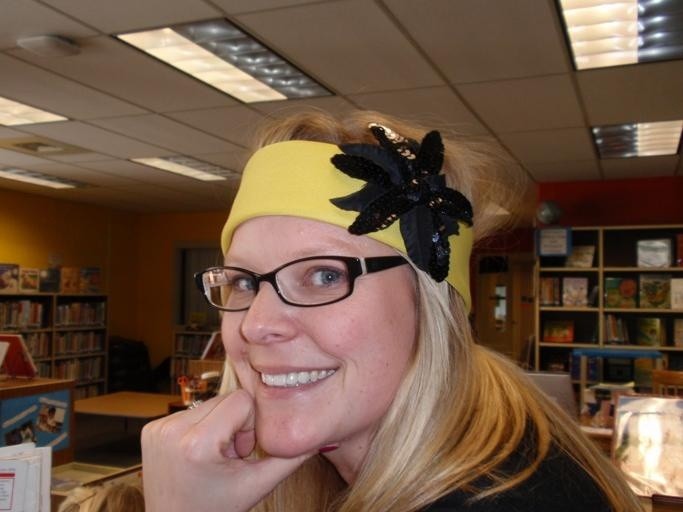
[142,106,646,512]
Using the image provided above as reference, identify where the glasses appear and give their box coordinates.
[194,256,409,311]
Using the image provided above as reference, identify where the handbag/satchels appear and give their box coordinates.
[612,398,683,498]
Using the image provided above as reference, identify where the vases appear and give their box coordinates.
[182,389,194,406]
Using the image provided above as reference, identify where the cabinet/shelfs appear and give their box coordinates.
[0,293,109,401]
[170,329,219,395]
[535,223,683,416]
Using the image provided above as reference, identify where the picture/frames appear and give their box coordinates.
[19,268,39,293]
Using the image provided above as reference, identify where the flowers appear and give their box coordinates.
[177,373,207,391]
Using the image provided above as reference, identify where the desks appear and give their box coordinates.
[0,376,75,468]
[73,391,182,419]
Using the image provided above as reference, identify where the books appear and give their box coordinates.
[0,262,106,399]
[539,234,683,427]
